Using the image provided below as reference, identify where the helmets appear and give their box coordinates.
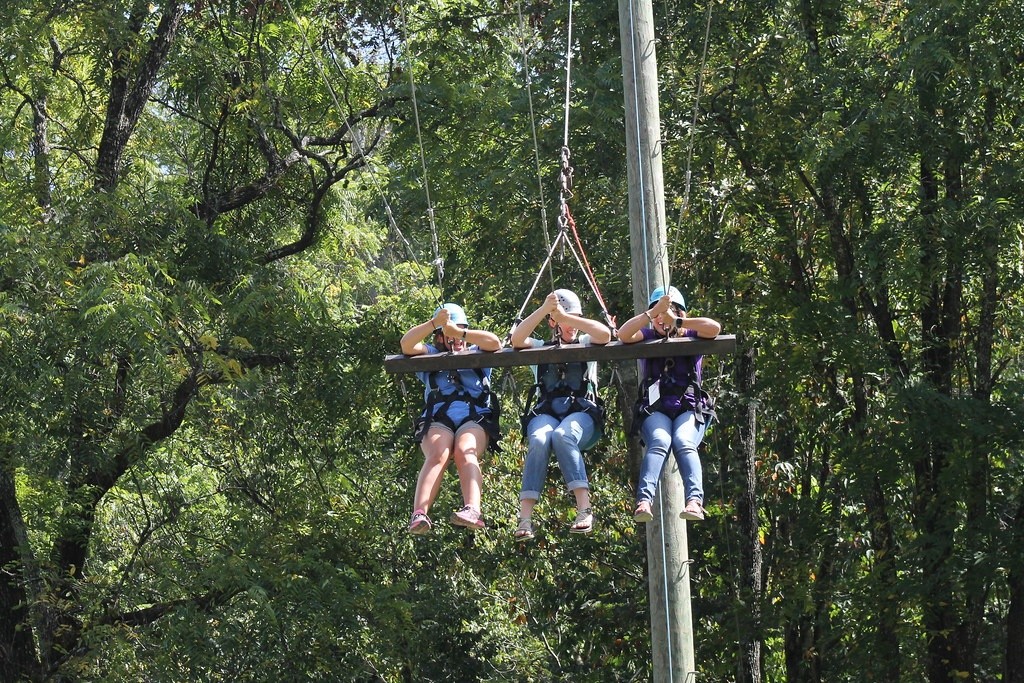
[648,285,686,313]
[545,289,583,317]
[433,303,469,333]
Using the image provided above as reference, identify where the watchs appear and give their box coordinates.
[674,317,683,330]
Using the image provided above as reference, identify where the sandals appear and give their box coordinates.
[569,508,593,533]
[515,519,534,542]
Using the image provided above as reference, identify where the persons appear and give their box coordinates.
[401,303,501,535]
[618,285,722,521]
[512,288,611,541]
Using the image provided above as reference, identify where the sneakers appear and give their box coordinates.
[679,500,708,520]
[633,501,653,522]
[410,509,432,534]
[451,505,485,530]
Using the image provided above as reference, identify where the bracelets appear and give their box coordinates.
[430,320,435,329]
[462,328,467,342]
[644,311,652,322]
